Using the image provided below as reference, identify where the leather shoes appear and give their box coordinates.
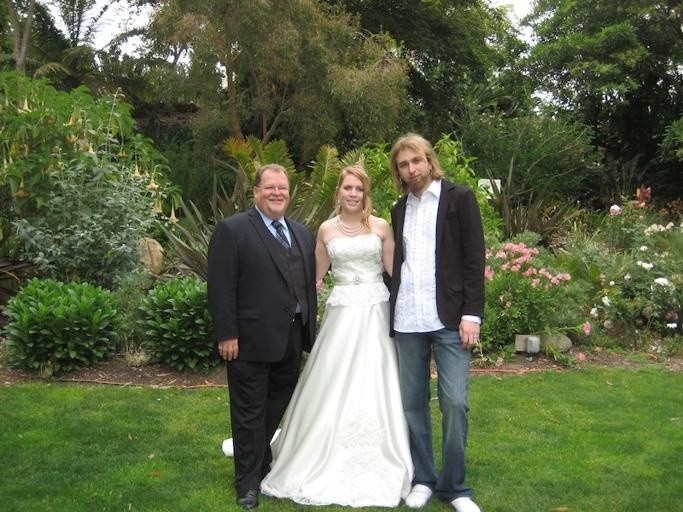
[238,493,258,509]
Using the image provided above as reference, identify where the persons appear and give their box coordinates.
[208,164,317,510]
[259,165,414,512]
[389,133,486,512]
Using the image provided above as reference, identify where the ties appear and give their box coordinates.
[272,221,290,246]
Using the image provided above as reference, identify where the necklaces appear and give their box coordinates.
[336,214,368,237]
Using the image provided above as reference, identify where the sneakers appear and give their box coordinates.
[452,497,480,512]
[405,484,433,509]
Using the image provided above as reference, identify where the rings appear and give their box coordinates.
[473,336,479,340]
